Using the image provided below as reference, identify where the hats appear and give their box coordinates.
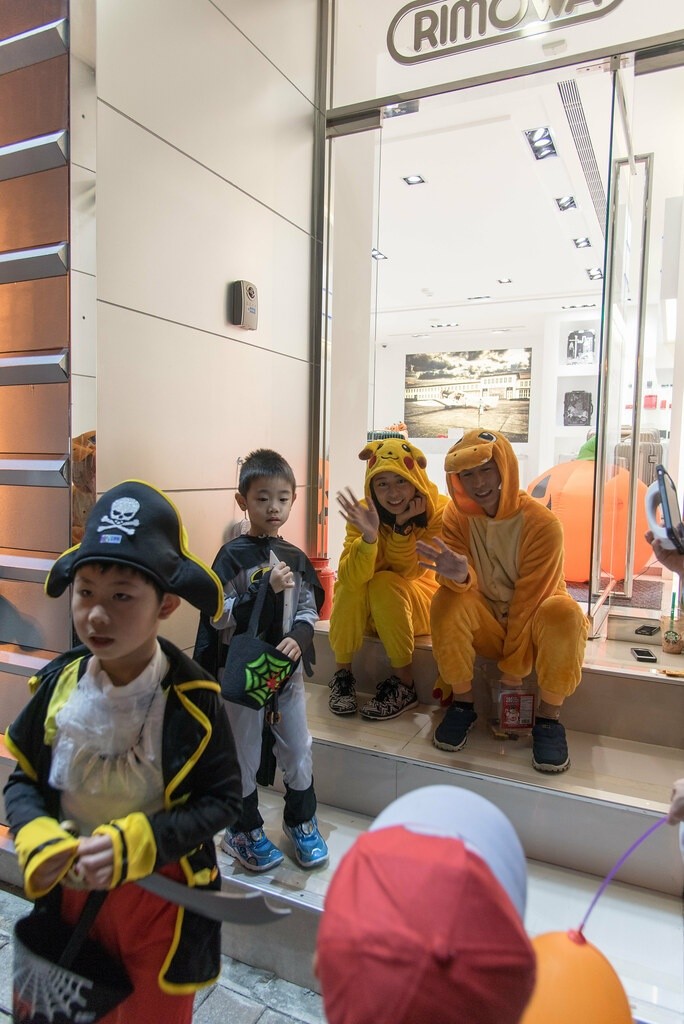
[315,783,536,1024]
[45,479,224,623]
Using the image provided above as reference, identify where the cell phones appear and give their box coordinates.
[656,464,683,554]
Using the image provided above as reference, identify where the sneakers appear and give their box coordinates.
[432,703,479,753]
[219,820,283,873]
[359,674,419,719]
[281,814,329,868]
[531,719,570,772]
[326,668,358,714]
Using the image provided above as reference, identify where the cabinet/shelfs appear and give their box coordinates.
[539,303,627,479]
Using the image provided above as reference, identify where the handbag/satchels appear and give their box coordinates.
[220,572,297,709]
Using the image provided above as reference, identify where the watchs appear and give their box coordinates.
[393,522,413,536]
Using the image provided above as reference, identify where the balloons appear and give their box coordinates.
[516,930,635,1024]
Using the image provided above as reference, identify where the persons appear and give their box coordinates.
[192,450,330,870]
[414,429,590,771]
[327,438,452,720]
[645,519,684,825]
[3,481,245,1024]
[313,782,538,1024]
